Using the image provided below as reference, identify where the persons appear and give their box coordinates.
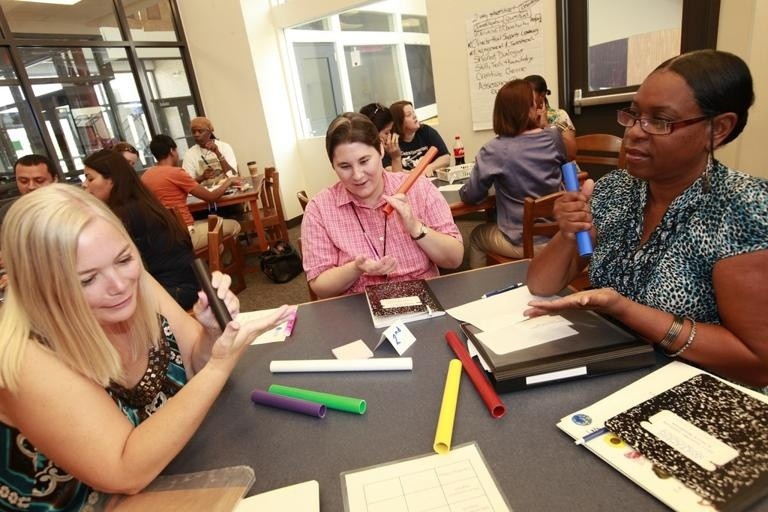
[81,150,202,310]
[142,135,244,265]
[524,50,768,388]
[111,142,138,169]
[181,117,244,222]
[458,79,569,269]
[301,113,464,299]
[359,103,403,173]
[0,184,294,510]
[523,74,579,174]
[14,154,59,195]
[390,102,450,178]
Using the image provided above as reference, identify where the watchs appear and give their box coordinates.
[410,222,427,241]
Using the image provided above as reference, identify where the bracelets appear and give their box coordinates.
[660,317,684,349]
[664,310,697,357]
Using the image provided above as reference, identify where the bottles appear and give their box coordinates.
[453,136,465,166]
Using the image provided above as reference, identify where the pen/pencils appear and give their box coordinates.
[199,154,211,169]
[480,282,523,300]
[574,425,608,446]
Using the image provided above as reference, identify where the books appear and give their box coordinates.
[556,359,768,511]
[604,373,768,511]
[459,308,655,381]
[362,278,446,329]
[104,464,257,511]
[482,351,658,394]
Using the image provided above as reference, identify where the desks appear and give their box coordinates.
[89,253,768,511]
[419,162,494,218]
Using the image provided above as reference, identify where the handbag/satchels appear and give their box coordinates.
[258,239,302,284]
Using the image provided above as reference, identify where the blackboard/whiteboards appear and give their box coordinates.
[465,2,560,132]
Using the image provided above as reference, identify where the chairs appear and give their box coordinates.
[298,189,321,302]
[136,160,292,295]
[486,189,572,269]
[568,130,628,291]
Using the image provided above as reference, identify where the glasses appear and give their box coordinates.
[617,108,709,135]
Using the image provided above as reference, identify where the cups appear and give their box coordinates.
[247,163,259,177]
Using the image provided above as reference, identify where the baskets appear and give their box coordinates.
[435,163,474,184]
